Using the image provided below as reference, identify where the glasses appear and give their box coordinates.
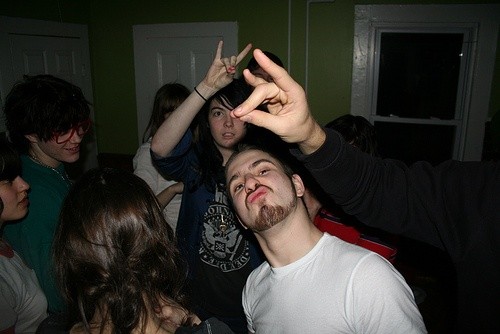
[56,122,90,145]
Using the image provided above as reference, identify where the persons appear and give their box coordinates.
[0,73,92,334]
[113,37,379,334]
[222,147,428,334]
[36,167,235,334]
[229,48,500,334]
